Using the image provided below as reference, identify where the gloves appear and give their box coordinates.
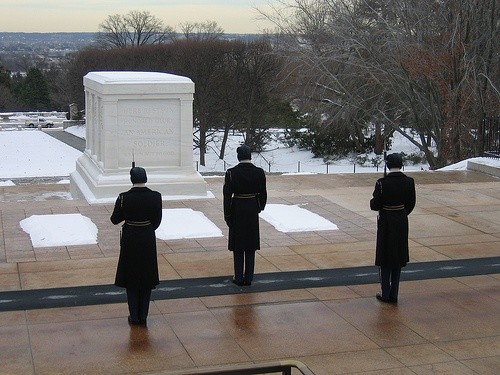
[225,216,232,228]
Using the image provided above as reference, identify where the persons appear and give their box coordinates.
[370,153,416,304]
[223,147,267,286]
[110,166,162,325]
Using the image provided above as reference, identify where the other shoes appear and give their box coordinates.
[128,316,146,325]
[233,276,251,285]
[376,292,383,301]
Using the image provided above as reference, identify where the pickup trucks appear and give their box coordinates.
[25,117,54,128]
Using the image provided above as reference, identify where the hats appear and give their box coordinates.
[386,153,404,170]
[237,144,251,161]
[130,167,147,184]
